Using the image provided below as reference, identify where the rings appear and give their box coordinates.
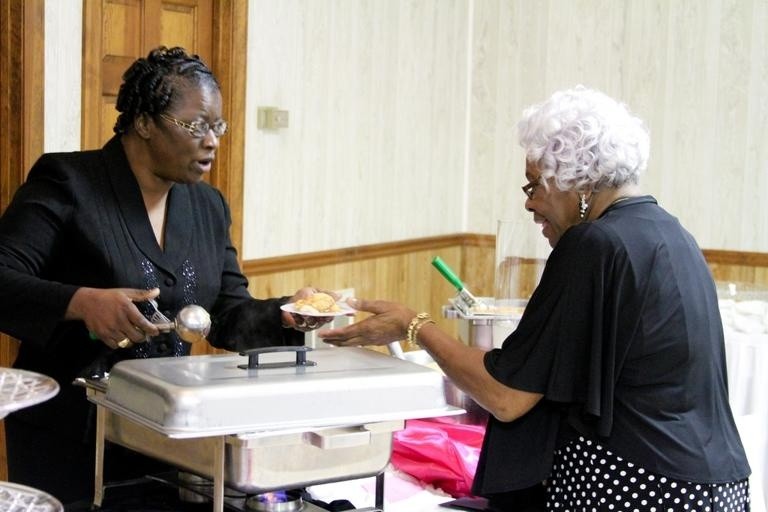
[118,338,130,349]
[295,319,304,331]
[305,320,320,331]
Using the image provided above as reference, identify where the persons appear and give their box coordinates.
[316,83,752,512]
[0,42,357,512]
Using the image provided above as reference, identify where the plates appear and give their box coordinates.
[280,301,358,317]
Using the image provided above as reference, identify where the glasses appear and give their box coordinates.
[520,181,542,197]
[161,113,227,138]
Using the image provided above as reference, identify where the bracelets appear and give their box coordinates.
[412,320,436,349]
[407,311,431,349]
